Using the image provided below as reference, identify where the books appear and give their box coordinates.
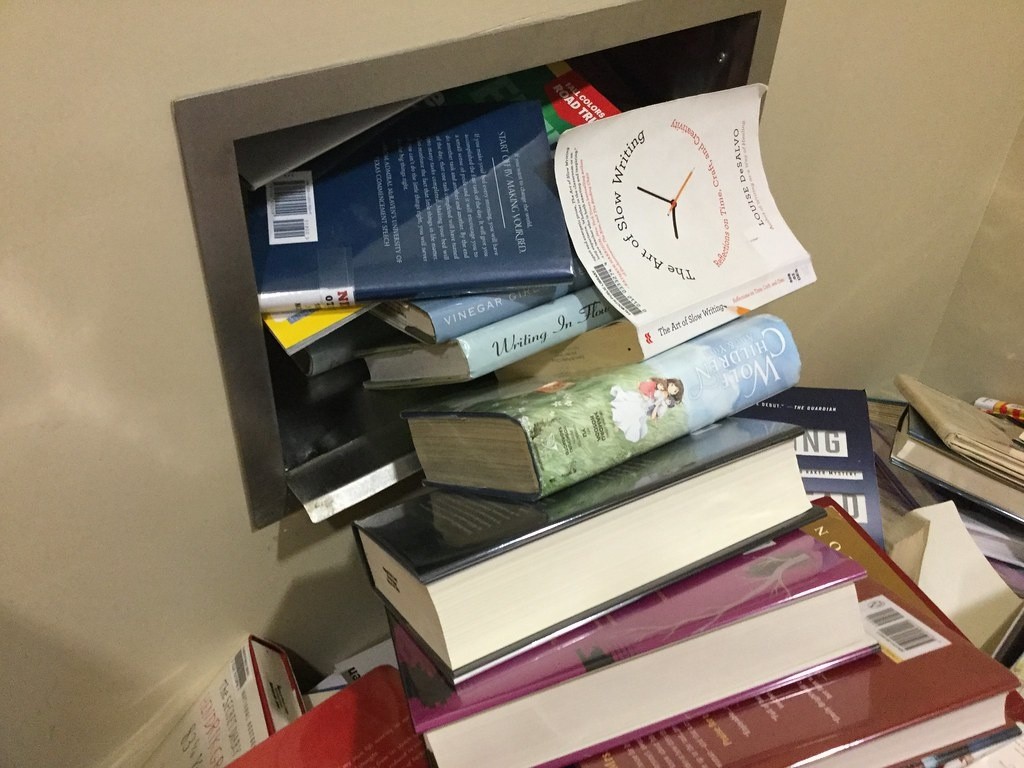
[141,60,1024,768]
